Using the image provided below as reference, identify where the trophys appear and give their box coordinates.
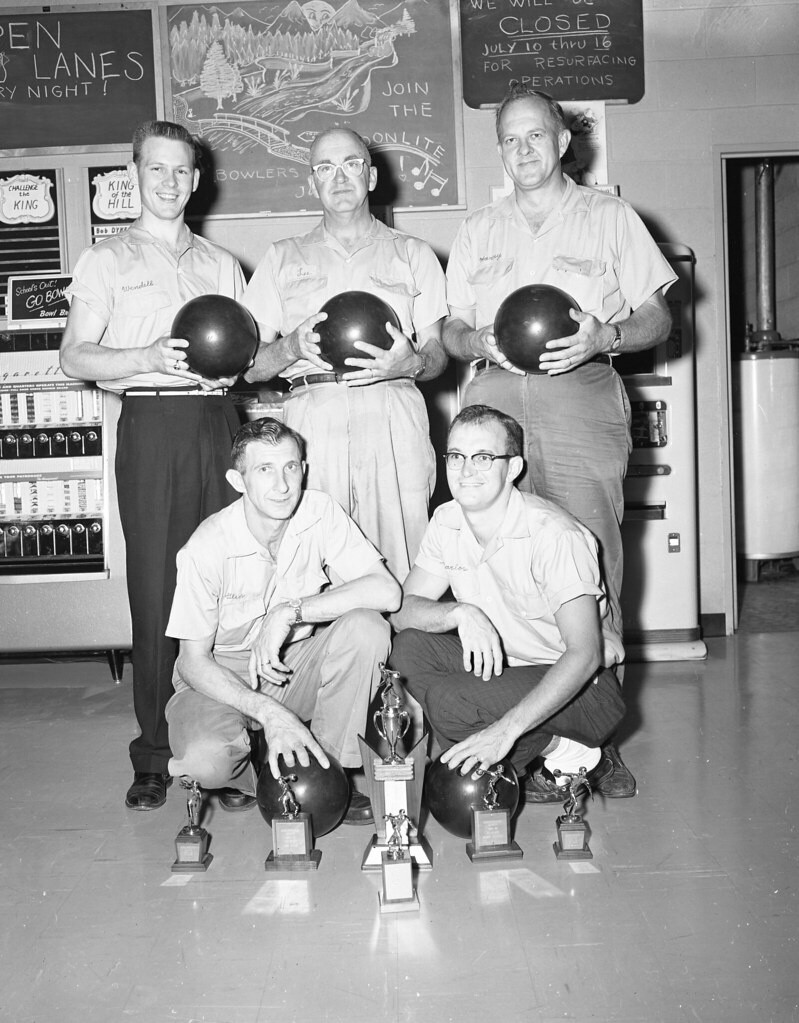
[265,771,323,870]
[465,764,524,863]
[358,659,436,874]
[551,763,596,860]
[170,780,214,871]
[378,809,423,914]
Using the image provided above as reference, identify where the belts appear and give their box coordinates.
[476,352,610,370]
[125,387,228,395]
[292,373,343,387]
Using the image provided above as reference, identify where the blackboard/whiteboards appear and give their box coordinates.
[459,0,645,111]
[0,2,164,158]
[8,274,73,321]
[157,0,467,220]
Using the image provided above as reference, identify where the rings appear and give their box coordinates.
[567,359,572,366]
[369,369,374,377]
[261,660,271,666]
[473,755,480,762]
[174,360,179,369]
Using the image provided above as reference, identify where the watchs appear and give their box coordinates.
[410,352,426,380]
[290,596,304,624]
[607,322,621,353]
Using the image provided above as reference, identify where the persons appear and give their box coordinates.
[395,404,635,805]
[237,128,451,633]
[165,416,404,826]
[439,90,678,794]
[59,121,253,815]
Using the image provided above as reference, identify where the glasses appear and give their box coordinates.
[311,157,370,182]
[442,451,512,471]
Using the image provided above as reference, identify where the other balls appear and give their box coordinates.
[313,291,400,375]
[256,750,350,838]
[172,295,258,379]
[494,284,582,375]
[426,746,520,839]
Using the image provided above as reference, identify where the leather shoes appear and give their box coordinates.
[218,789,257,811]
[593,742,633,797]
[342,789,373,825]
[125,770,173,811]
[519,757,613,800]
